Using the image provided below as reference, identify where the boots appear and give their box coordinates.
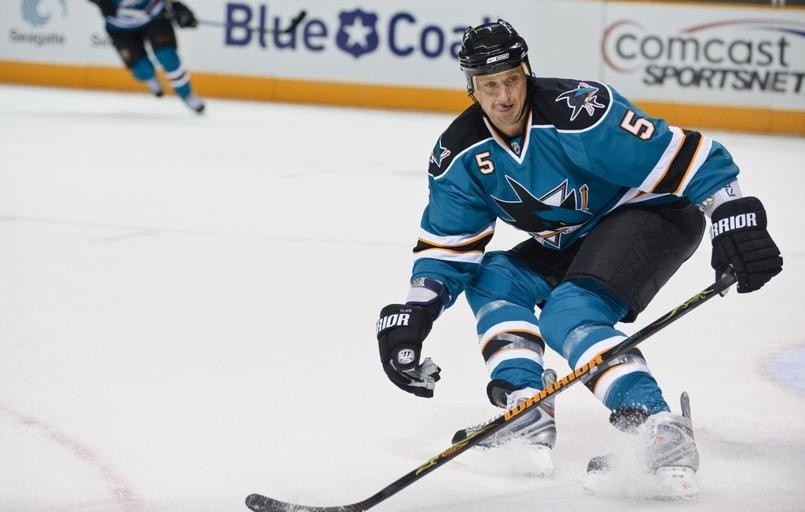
[452,369,556,448]
[587,392,699,472]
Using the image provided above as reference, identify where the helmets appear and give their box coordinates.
[459,19,532,93]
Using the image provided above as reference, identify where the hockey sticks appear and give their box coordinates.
[194,12,308,34]
[246,274,738,511]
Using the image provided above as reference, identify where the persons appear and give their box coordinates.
[91,1,207,117]
[377,20,783,498]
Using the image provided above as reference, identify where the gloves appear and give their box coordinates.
[376,304,441,398]
[709,197,783,297]
